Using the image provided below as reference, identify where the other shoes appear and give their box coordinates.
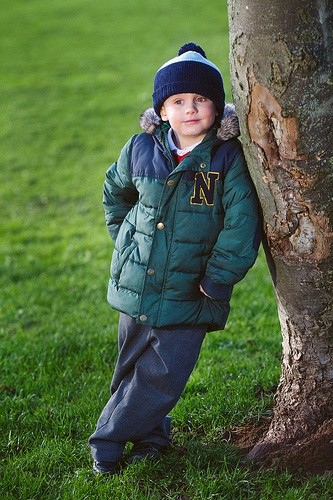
[125,442,166,462]
[94,457,123,475]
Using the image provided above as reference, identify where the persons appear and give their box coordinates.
[87,42,262,478]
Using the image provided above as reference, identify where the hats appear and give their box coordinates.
[152,42,225,121]
